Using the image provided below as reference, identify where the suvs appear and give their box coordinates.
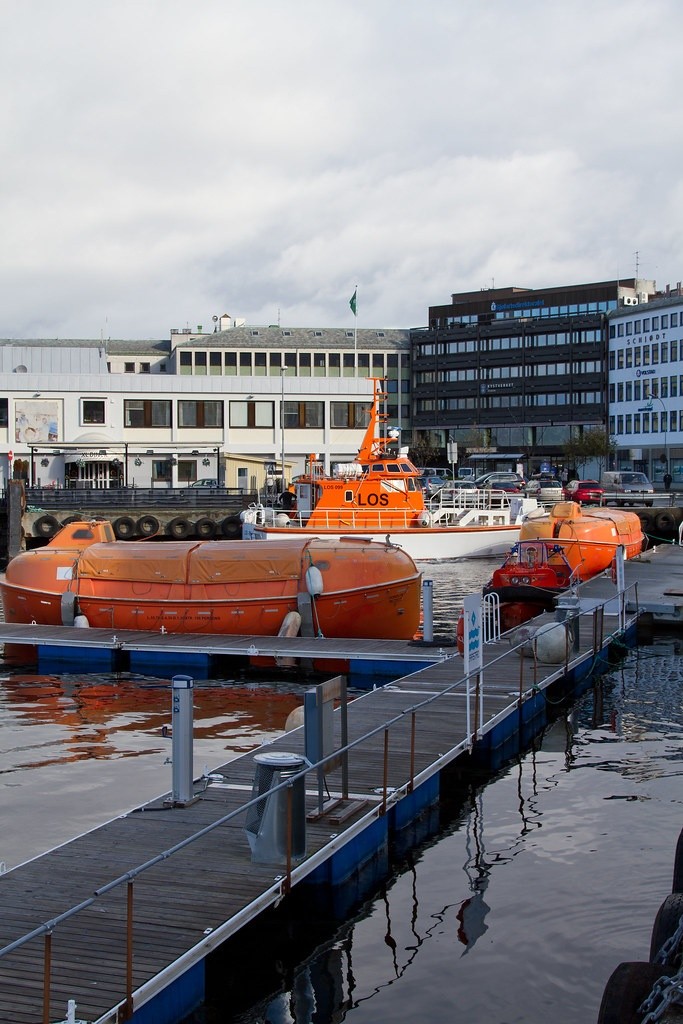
[473,471,526,492]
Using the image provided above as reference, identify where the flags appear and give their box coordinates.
[349,290,356,316]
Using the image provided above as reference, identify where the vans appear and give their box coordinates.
[458,467,487,480]
[416,467,454,481]
[601,471,653,508]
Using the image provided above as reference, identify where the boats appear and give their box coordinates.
[3,517,424,640]
[519,499,641,585]
[239,373,556,560]
[483,536,574,633]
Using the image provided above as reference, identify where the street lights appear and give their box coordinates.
[648,393,667,486]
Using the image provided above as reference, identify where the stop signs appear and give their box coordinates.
[8,451,13,461]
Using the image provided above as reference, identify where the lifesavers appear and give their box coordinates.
[612,554,616,584]
[457,609,464,658]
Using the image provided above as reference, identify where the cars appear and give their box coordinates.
[480,481,520,507]
[408,476,428,499]
[420,477,445,500]
[520,479,567,505]
[562,479,605,507]
[437,480,479,506]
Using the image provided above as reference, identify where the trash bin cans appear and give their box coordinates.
[242,751,315,865]
[555,604,581,651]
